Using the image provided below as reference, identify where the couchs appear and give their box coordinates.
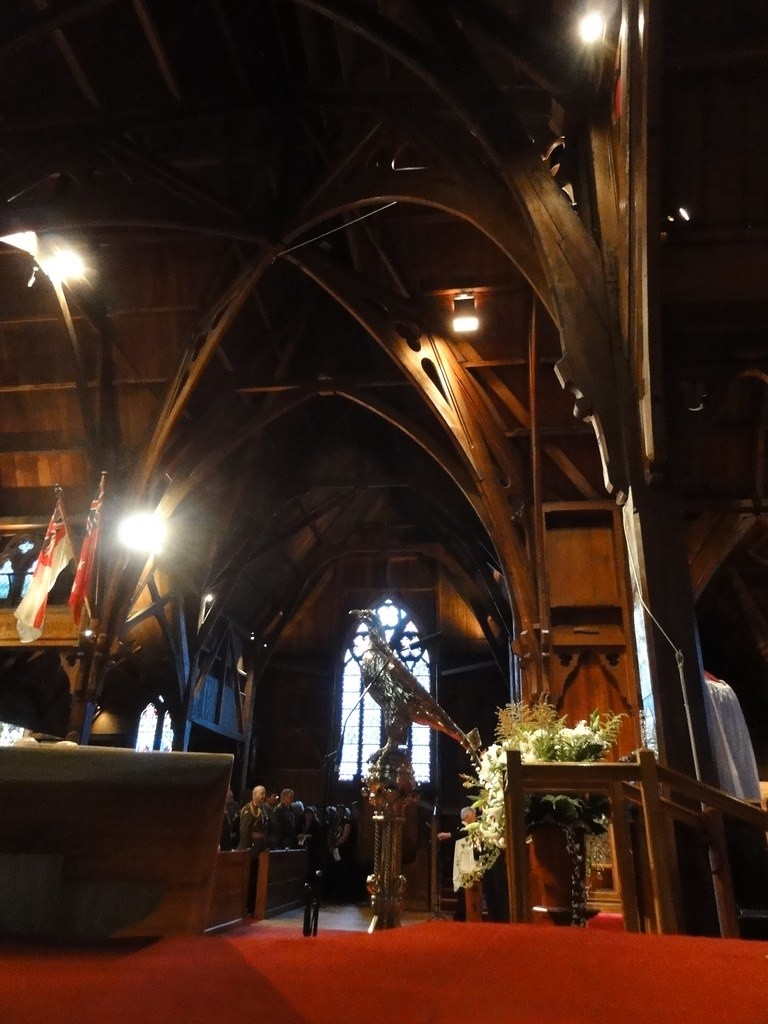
[0,742,235,940]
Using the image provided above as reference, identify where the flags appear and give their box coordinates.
[69,474,108,623]
[13,500,75,643]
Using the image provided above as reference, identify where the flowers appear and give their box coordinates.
[458,693,630,885]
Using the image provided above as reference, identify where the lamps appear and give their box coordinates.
[452,297,481,332]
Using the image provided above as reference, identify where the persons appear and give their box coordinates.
[218,785,508,923]
